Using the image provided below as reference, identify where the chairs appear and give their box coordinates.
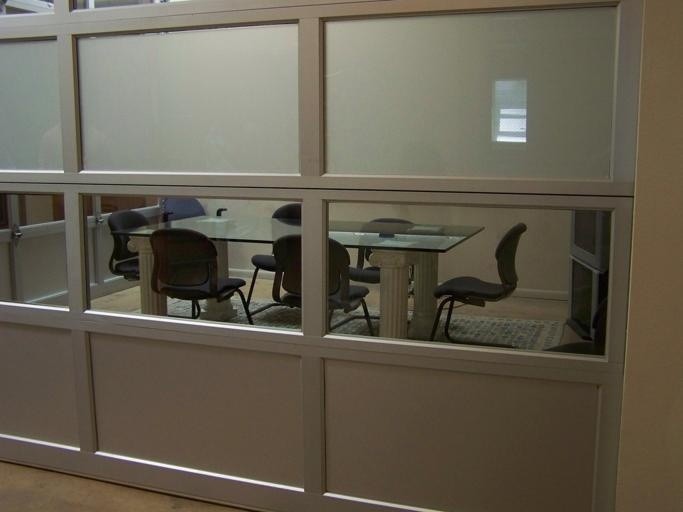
[149,229,255,327]
[345,217,416,321]
[271,233,376,336]
[106,211,154,283]
[429,222,527,349]
[245,202,302,317]
[156,197,208,224]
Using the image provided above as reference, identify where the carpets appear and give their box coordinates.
[126,295,568,352]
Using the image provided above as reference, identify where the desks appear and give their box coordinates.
[109,214,486,340]
